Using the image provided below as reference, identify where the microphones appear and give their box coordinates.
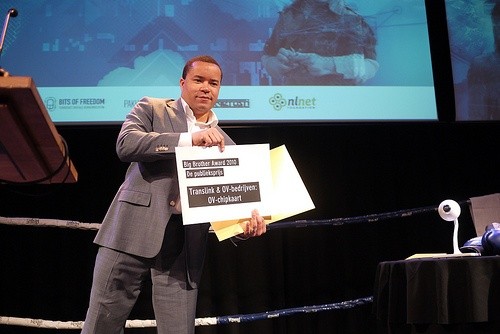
[0,8,18,54]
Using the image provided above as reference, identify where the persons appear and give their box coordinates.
[465,4,500,120]
[80,54,268,334]
[259,0,379,86]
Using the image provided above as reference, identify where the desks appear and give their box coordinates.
[372,256,500,334]
